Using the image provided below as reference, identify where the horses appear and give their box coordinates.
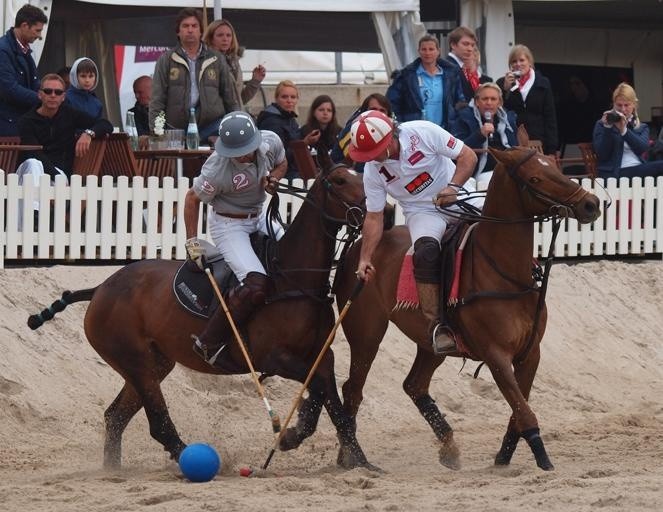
[26,143,399,477]
[328,123,604,470]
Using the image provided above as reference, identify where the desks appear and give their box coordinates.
[2,143,42,175]
[132,148,214,178]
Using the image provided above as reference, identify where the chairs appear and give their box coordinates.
[1,136,20,174]
[527,139,544,155]
[290,141,319,179]
[577,141,599,188]
[103,133,140,178]
[74,132,109,178]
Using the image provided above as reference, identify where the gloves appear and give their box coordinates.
[183,235,209,272]
[262,174,280,196]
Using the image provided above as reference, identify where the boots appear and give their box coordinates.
[410,235,458,353]
[191,271,274,373]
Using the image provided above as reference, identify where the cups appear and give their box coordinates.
[162,128,183,150]
[148,137,161,151]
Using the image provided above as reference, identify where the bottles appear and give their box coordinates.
[185,108,200,151]
[126,110,139,152]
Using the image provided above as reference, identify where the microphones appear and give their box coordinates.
[484,110,494,139]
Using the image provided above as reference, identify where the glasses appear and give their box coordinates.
[38,87,66,96]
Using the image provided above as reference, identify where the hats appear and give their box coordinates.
[213,110,262,158]
[346,109,394,163]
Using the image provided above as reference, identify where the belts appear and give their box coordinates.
[212,209,263,220]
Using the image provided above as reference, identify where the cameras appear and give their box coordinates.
[513,71,522,77]
[606,111,620,124]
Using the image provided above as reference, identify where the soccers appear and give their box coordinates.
[178,443,220,481]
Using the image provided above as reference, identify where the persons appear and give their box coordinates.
[328,91,393,172]
[300,94,345,175]
[146,7,243,150]
[183,108,291,375]
[384,25,493,128]
[254,80,304,186]
[494,42,559,156]
[346,109,491,351]
[590,80,662,187]
[0,3,115,271]
[124,74,154,139]
[447,80,520,193]
[200,18,266,115]
